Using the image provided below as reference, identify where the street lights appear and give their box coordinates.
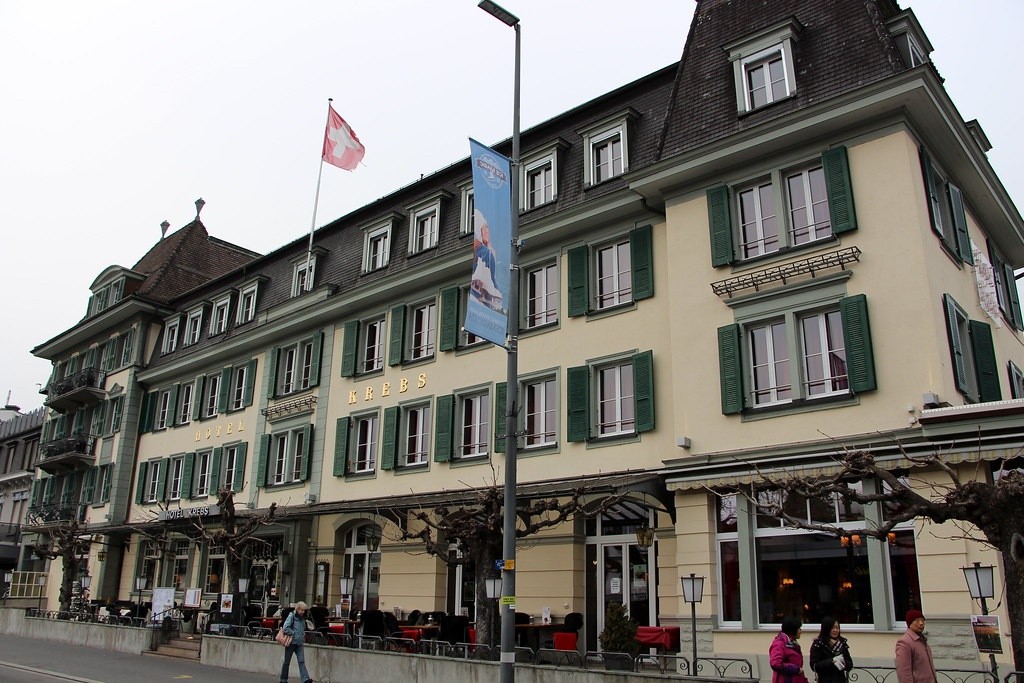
[36,573,48,617]
[679,573,705,676]
[79,571,93,622]
[476,0,522,683]
[338,575,357,650]
[959,561,1000,683]
[135,574,148,627]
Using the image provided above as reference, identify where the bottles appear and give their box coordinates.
[529,614,534,624]
[332,608,336,617]
[356,611,361,622]
[428,615,434,626]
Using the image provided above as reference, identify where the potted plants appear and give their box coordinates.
[598,599,635,670]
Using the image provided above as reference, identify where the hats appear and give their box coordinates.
[905,610,925,627]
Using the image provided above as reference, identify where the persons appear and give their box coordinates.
[173,602,183,618]
[769,615,810,683]
[280,601,313,683]
[809,616,853,683]
[894,608,938,683]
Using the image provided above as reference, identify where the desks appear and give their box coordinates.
[398,626,440,641]
[635,625,680,674]
[256,616,283,641]
[514,621,572,665]
[325,619,359,647]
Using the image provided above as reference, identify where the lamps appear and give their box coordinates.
[98,549,107,564]
[844,581,851,588]
[840,532,898,547]
[783,577,793,585]
[635,492,655,547]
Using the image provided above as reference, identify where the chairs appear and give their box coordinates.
[88,597,584,654]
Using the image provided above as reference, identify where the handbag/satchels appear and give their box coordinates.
[275,611,294,647]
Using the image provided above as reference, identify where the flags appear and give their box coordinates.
[321,105,365,173]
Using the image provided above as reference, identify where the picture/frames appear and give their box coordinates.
[220,594,234,613]
[151,587,175,620]
[184,587,203,607]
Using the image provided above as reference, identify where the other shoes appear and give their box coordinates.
[304,679,312,683]
